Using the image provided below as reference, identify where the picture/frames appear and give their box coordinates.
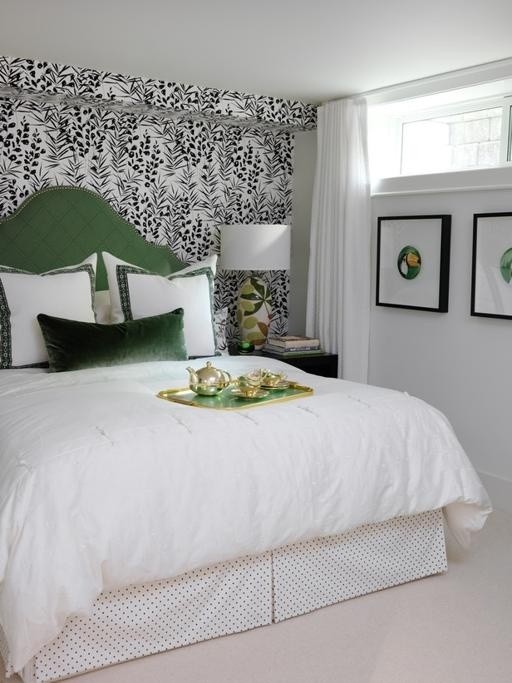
[470,212,512,320]
[376,213,452,314]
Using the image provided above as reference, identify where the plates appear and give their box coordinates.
[230,388,269,400]
[262,381,290,391]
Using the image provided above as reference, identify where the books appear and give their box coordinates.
[263,335,324,357]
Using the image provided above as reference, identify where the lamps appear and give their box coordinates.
[221,224,291,355]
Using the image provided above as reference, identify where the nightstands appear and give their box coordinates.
[229,344,341,382]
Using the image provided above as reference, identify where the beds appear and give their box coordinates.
[0,182,495,683]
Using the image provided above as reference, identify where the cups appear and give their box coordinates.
[237,369,284,396]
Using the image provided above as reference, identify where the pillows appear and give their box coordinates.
[36,307,189,372]
[0,249,104,371]
[92,288,113,326]
[100,246,224,362]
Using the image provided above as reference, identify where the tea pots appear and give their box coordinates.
[185,361,231,396]
